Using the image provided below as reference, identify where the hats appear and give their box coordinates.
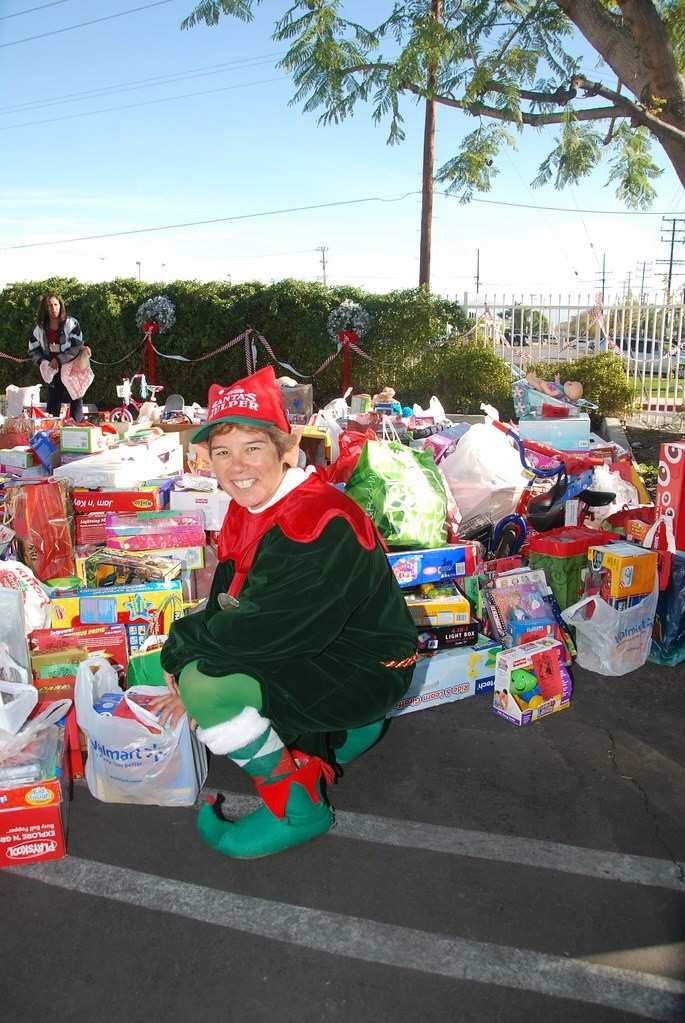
[189,365,292,444]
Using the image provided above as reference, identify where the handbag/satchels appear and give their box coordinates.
[343,415,449,548]
[75,656,209,806]
[308,387,379,484]
[3,475,77,582]
[560,568,659,677]
[589,500,656,542]
[527,525,620,657]
[127,594,186,689]
[412,395,446,423]
[642,515,684,666]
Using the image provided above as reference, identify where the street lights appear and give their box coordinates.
[135,261,141,282]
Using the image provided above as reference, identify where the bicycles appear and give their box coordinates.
[109,374,194,426]
[489,418,619,567]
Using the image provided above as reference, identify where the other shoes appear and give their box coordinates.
[335,716,385,763]
[197,750,335,859]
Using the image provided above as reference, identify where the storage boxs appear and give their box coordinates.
[0,385,685,867]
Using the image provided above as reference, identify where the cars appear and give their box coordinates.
[597,336,685,379]
[569,339,593,349]
[505,329,558,346]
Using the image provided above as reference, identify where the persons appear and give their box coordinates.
[149,365,419,860]
[27,293,84,424]
